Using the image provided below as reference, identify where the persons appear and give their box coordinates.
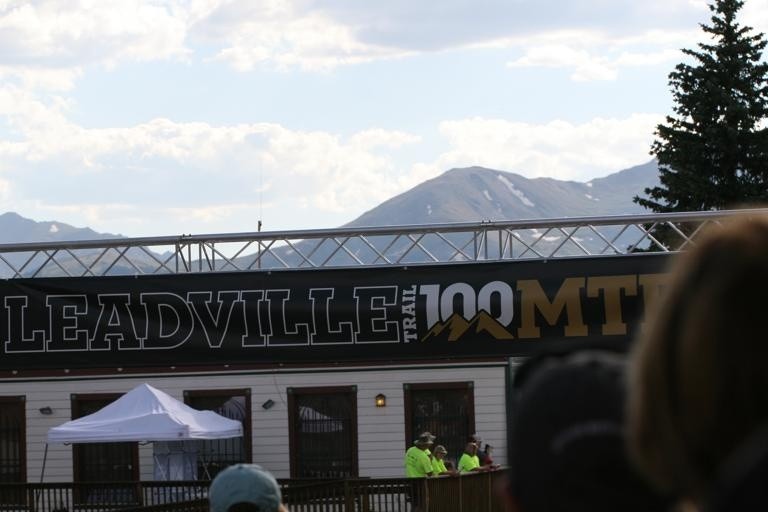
[404,431,500,477]
[623,206,768,510]
[501,352,675,512]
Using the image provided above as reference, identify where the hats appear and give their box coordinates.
[414,431,435,446]
